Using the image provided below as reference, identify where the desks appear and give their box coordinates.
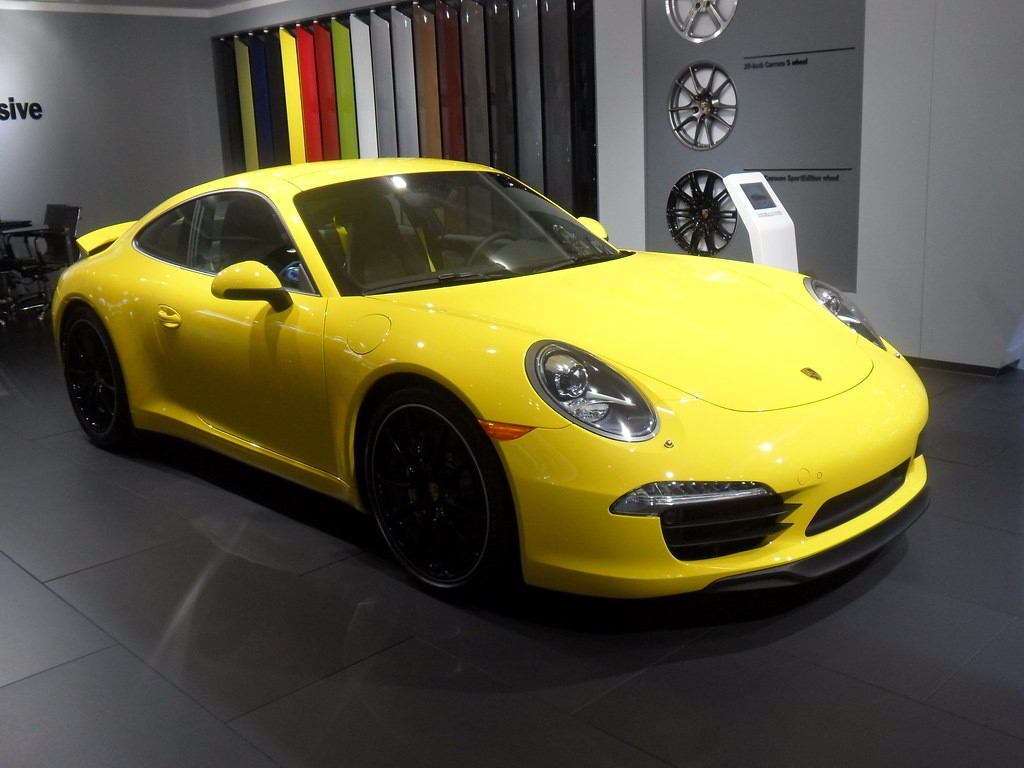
[0,216,68,310]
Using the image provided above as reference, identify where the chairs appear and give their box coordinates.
[345,193,426,281]
[221,201,295,290]
[3,203,83,321]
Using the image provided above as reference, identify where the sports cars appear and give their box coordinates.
[53,155,934,608]
